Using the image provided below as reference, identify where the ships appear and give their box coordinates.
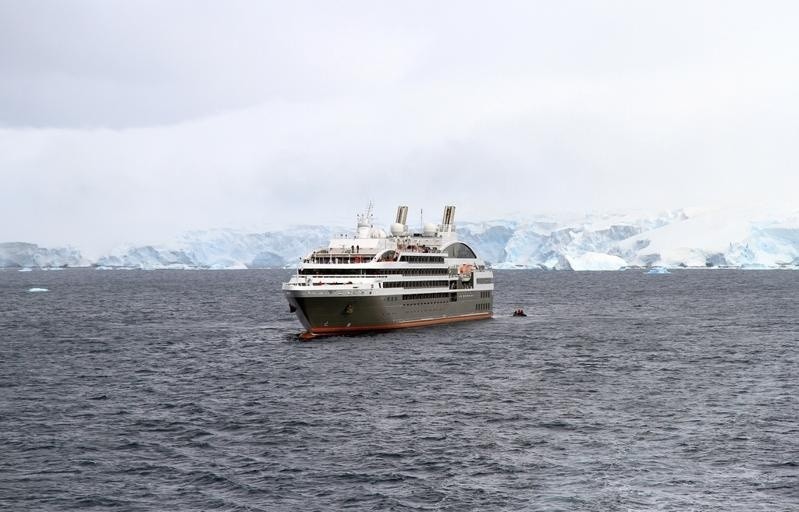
[283,202,496,339]
